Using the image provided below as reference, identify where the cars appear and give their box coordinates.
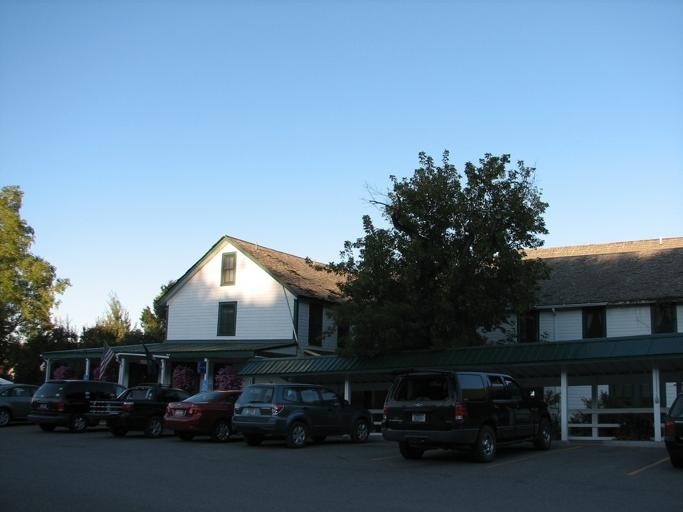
[662,392,683,468]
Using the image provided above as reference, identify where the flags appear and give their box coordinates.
[142,343,154,377]
[99,341,115,380]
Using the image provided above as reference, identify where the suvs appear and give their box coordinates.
[379,368,554,462]
